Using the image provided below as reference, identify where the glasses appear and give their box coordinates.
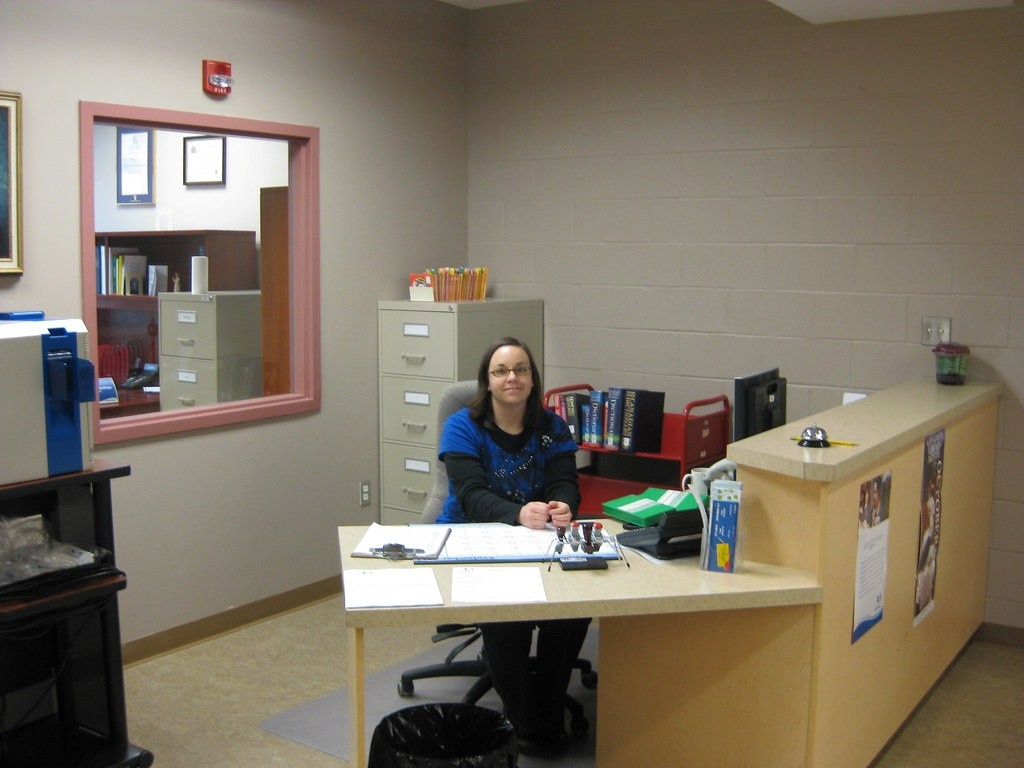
[488,365,533,377]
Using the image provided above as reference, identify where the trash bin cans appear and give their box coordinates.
[367,702,520,768]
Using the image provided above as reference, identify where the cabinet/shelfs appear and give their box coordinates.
[376,298,546,527]
[0,457,153,768]
[94,231,259,412]
[156,290,262,414]
[544,384,732,509]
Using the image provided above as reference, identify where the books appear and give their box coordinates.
[554,387,665,454]
[641,487,710,510]
[409,265,488,302]
[95,245,169,297]
[602,494,676,529]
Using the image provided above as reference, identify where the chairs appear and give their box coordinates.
[396,617,601,720]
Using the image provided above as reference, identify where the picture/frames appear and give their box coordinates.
[0,89,26,275]
[116,125,156,204]
[183,135,226,186]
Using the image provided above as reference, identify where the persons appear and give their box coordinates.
[872,478,890,526]
[859,490,870,528]
[436,335,581,759]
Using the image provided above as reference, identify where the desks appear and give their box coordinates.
[338,524,823,768]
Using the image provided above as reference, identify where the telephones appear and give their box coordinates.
[119,361,160,390]
[615,508,710,561]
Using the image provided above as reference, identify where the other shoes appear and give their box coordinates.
[503,707,569,758]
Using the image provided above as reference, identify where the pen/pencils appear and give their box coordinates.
[790,436,860,446]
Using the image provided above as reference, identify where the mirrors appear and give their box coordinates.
[78,98,322,447]
